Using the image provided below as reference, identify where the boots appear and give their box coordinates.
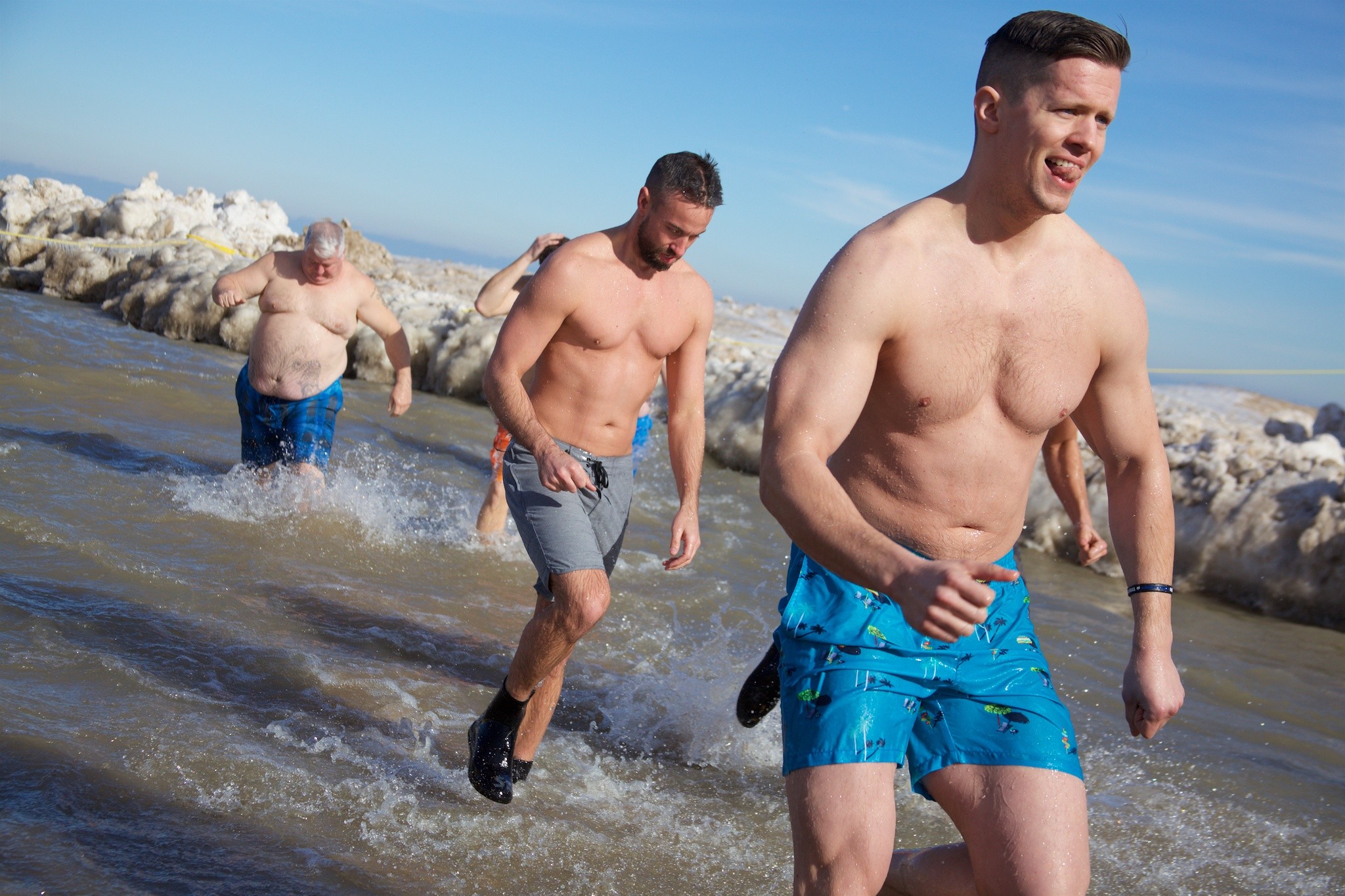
[510,759,533,783]
[468,676,537,803]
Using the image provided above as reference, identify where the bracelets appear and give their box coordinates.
[1127,583,1173,596]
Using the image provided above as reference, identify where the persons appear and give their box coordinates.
[212,221,412,485]
[474,233,571,533]
[736,413,1108,727]
[757,9,1184,896]
[631,357,667,476]
[469,151,725,804]
